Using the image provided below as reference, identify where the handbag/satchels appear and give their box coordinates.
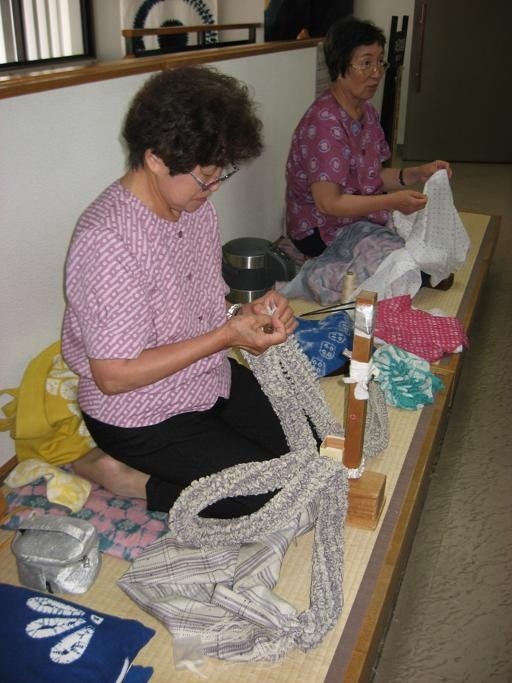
[0,340,98,465]
[11,516,100,594]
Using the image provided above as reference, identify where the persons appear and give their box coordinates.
[285,18,454,291]
[60,67,322,520]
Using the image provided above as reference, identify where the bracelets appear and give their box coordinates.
[398,167,409,186]
[226,303,242,320]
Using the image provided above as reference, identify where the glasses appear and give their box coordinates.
[351,62,390,74]
[189,160,240,192]
